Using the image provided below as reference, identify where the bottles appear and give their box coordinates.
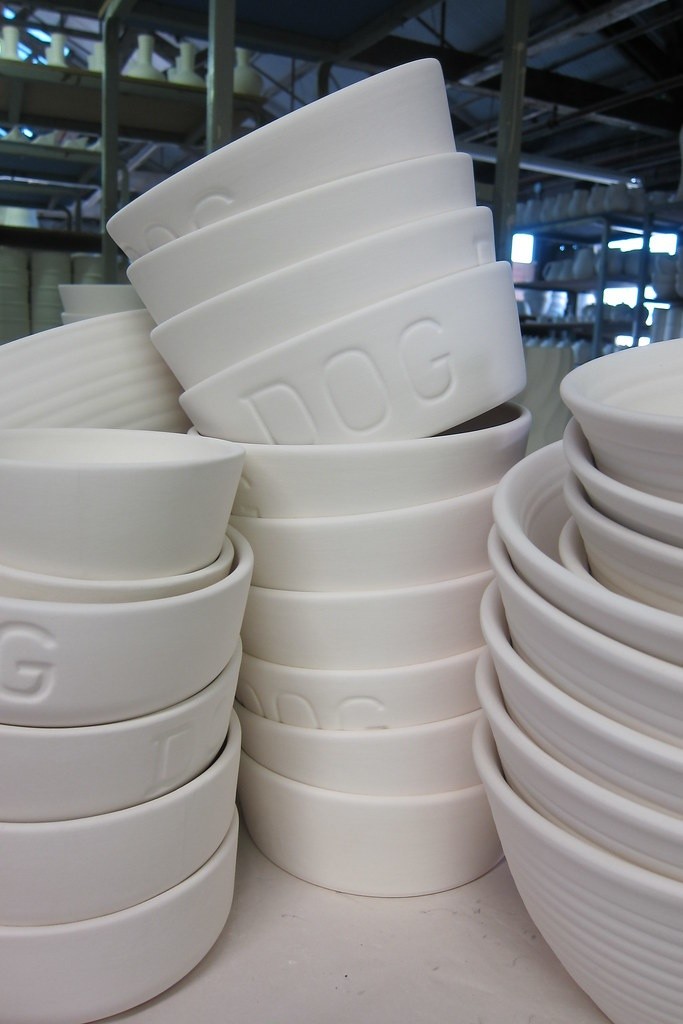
[130,34,207,86]
[234,46,265,95]
[2,25,102,73]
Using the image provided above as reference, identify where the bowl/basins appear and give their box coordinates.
[473,336,683,1024]
[0,284,257,1024]
[104,54,534,900]
[0,246,130,346]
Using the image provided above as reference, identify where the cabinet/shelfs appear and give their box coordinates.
[508,210,683,360]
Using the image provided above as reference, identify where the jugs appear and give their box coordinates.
[542,246,682,298]
[517,181,676,226]
